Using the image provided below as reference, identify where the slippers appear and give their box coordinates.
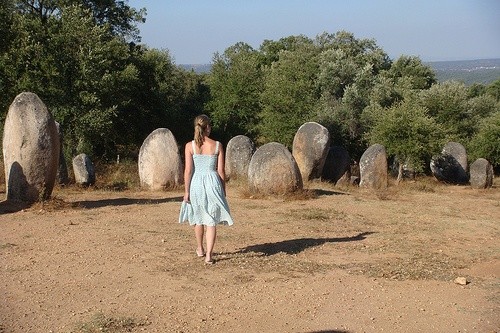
[205,259,215,264]
[196,248,204,256]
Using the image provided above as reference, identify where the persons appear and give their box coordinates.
[178,114,234,264]
